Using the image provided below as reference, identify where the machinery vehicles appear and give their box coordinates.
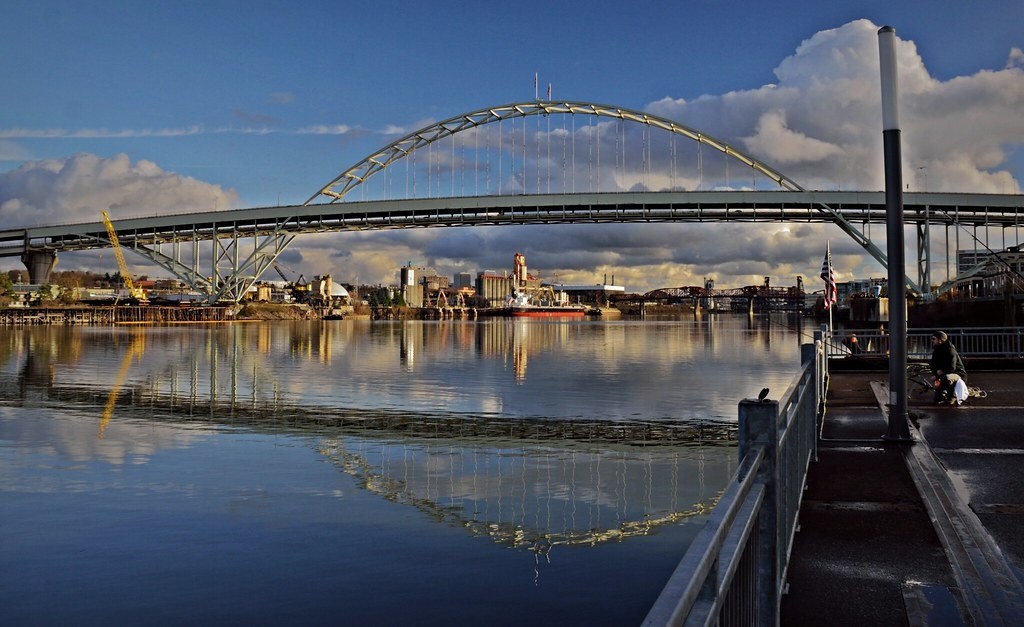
[262,253,313,302]
[99,209,150,306]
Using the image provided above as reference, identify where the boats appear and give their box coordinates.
[587,309,602,316]
[503,286,585,317]
[324,314,343,320]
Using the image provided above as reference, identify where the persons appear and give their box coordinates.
[930,331,968,404]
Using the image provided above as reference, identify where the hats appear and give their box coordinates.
[934,330,947,341]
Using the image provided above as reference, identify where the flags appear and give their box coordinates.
[820,242,837,309]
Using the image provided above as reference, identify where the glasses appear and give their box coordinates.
[931,336,935,340]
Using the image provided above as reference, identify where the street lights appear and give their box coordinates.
[99,253,103,287]
